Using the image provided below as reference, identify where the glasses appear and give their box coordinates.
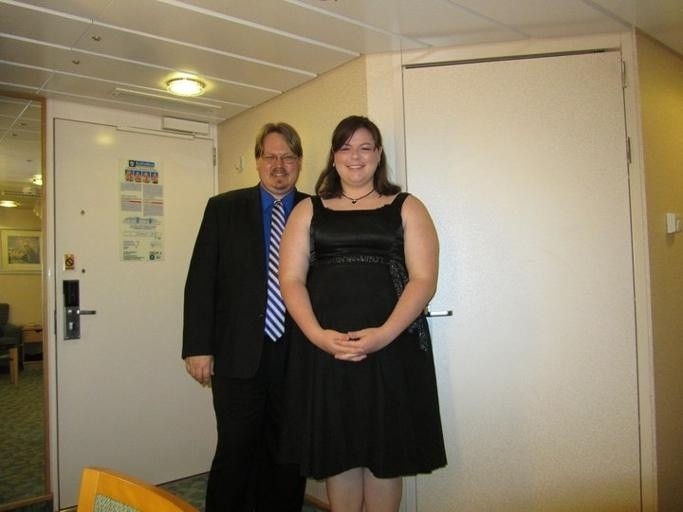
[262,152,298,163]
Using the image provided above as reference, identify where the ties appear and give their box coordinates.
[264,200,286,343]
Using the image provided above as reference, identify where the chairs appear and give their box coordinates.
[77,467,202,512]
[0,303,20,384]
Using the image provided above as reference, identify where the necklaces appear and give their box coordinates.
[340,188,376,204]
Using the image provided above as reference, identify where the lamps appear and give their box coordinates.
[165,78,206,96]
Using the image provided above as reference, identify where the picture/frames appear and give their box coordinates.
[0,227,41,275]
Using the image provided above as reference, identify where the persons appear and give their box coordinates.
[182,121,313,511]
[277,115,448,512]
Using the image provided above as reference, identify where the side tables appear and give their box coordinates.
[22,326,43,370]
